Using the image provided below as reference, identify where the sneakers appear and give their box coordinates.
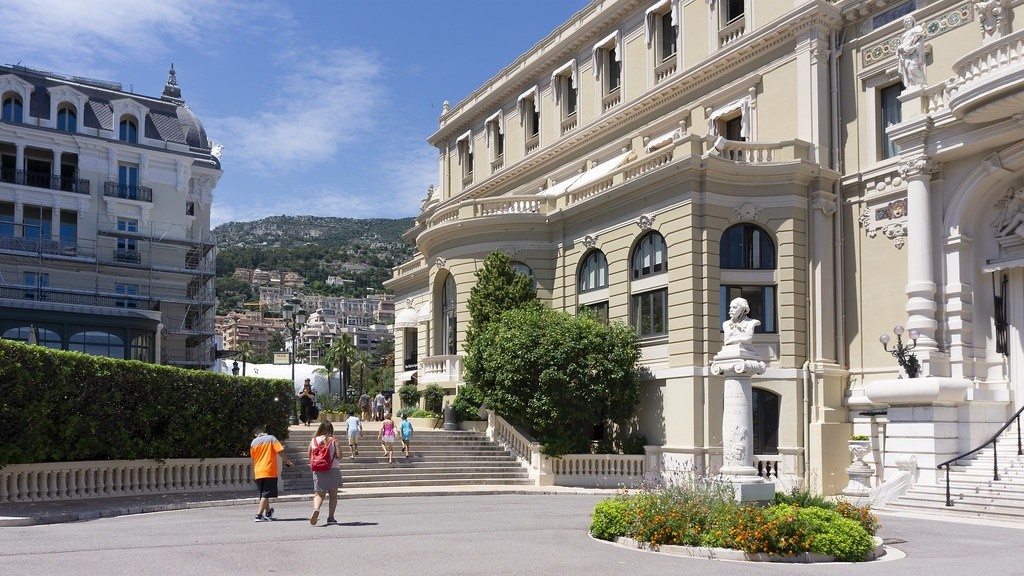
[265,506,275,520]
[310,509,320,525]
[326,517,339,526]
[253,513,267,523]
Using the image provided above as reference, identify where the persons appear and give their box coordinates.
[377,413,399,464]
[250,426,294,522]
[897,13,930,88]
[358,388,391,421]
[722,298,762,346]
[308,420,343,525]
[345,409,364,459]
[400,413,414,458]
[405,295,417,309]
[297,377,320,426]
[890,200,905,219]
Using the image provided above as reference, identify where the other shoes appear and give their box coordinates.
[388,460,392,464]
[383,451,388,457]
[402,447,405,452]
[349,455,354,459]
[406,455,409,458]
[303,422,310,427]
[355,450,358,455]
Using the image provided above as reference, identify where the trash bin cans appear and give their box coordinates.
[443,405,457,430]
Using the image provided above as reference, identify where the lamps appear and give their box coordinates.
[879,326,920,378]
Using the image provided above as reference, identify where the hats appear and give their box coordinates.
[304,377,311,382]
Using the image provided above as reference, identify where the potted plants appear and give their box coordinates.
[848,435,871,460]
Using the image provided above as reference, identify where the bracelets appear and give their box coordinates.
[289,461,293,465]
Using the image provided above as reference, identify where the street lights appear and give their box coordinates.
[281,292,309,425]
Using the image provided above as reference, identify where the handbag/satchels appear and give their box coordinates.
[311,405,319,420]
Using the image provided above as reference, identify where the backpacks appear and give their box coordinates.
[309,436,335,473]
